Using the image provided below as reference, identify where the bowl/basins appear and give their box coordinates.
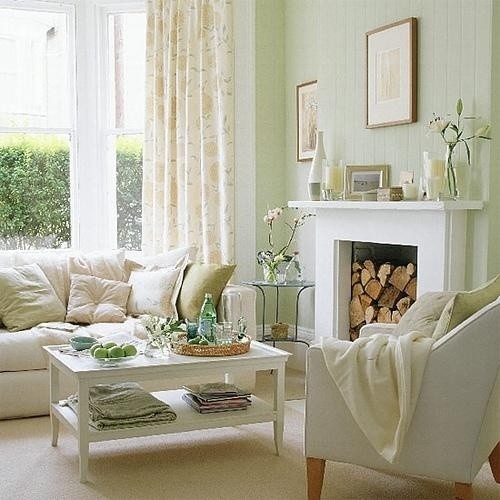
[70,337,98,351]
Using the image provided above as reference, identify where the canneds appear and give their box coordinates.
[390,187,402,200]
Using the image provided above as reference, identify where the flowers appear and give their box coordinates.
[257,204,317,277]
[424,98,493,196]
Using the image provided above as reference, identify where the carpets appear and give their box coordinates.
[253,367,306,403]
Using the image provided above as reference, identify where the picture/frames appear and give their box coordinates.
[295,80,317,162]
[364,17,418,129]
[344,164,390,200]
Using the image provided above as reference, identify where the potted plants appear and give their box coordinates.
[144,310,184,358]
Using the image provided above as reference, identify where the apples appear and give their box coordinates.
[107,346,125,358]
[121,344,137,356]
[89,344,103,357]
[103,342,116,349]
[93,347,107,358]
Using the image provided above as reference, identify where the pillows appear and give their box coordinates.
[66,274,133,323]
[177,262,236,323]
[127,254,189,321]
[392,291,454,338]
[431,275,500,342]
[1,263,66,332]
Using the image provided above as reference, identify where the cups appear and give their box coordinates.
[376,187,390,202]
[402,184,417,201]
[390,185,402,201]
[321,176,334,200]
[185,318,198,342]
[321,159,346,201]
[276,274,286,284]
[402,183,417,201]
[421,151,448,201]
[213,322,233,346]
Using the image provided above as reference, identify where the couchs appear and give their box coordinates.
[1,247,258,420]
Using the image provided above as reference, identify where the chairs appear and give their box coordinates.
[305,275,500,500]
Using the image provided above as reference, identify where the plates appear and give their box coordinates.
[88,353,138,368]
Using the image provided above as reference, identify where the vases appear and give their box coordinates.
[308,131,328,201]
[438,141,462,200]
[263,264,289,284]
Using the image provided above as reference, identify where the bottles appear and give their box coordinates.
[199,293,216,342]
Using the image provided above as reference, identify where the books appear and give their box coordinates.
[182,383,252,414]
